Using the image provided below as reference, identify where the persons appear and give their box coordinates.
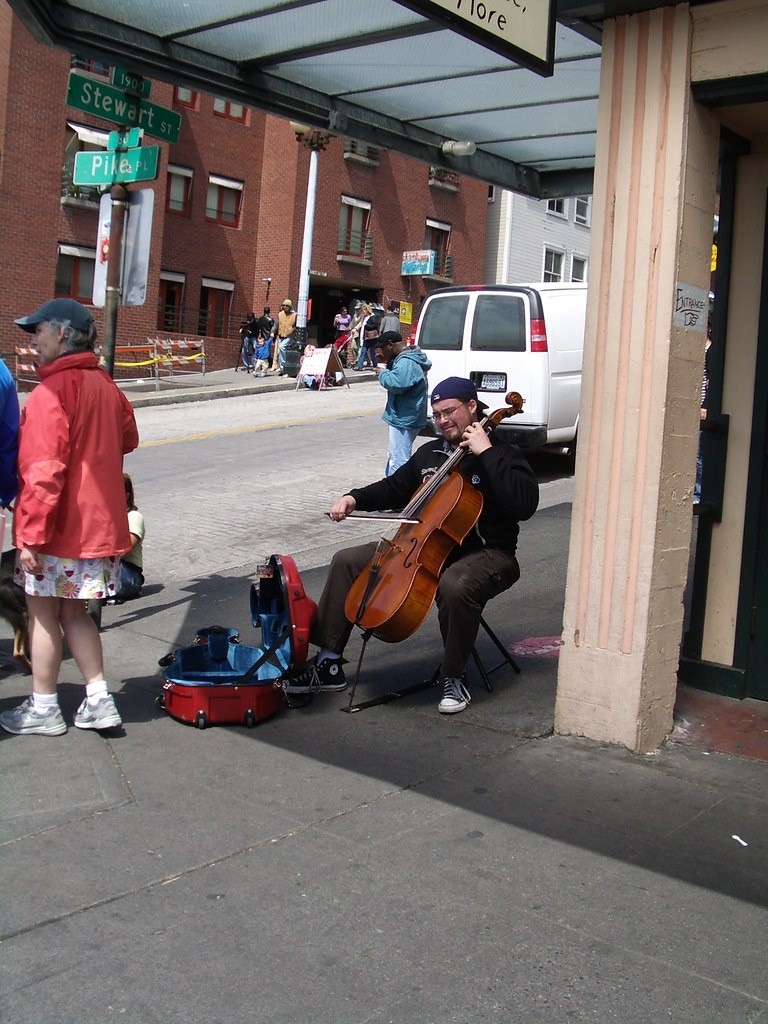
[261,307,277,369]
[239,312,258,371]
[374,331,432,476]
[0,299,145,736]
[300,339,341,388]
[333,304,400,371]
[693,313,714,515]
[281,377,539,714]
[252,336,273,377]
[270,300,297,375]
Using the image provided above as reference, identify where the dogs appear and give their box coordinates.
[0,548,33,672]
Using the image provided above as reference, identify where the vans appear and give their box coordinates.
[413,281,587,478]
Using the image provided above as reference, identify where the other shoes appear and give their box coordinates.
[240,365,283,377]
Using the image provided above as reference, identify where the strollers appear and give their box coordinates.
[332,331,354,369]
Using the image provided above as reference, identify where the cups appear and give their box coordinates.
[377,363,387,374]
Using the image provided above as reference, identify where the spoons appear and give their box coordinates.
[732,834,747,846]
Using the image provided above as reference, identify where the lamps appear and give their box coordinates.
[442,139,478,159]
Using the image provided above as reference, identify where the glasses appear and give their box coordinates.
[282,305,289,307]
[432,402,466,422]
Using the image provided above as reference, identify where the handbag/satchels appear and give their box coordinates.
[365,329,379,340]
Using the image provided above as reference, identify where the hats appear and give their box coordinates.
[431,377,489,409]
[14,298,97,338]
[374,331,401,348]
[354,303,363,309]
[282,299,292,307]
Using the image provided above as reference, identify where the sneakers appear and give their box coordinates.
[438,669,471,713]
[0,695,67,736]
[73,694,122,729]
[284,652,349,692]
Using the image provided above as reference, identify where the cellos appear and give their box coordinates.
[339,391,524,714]
